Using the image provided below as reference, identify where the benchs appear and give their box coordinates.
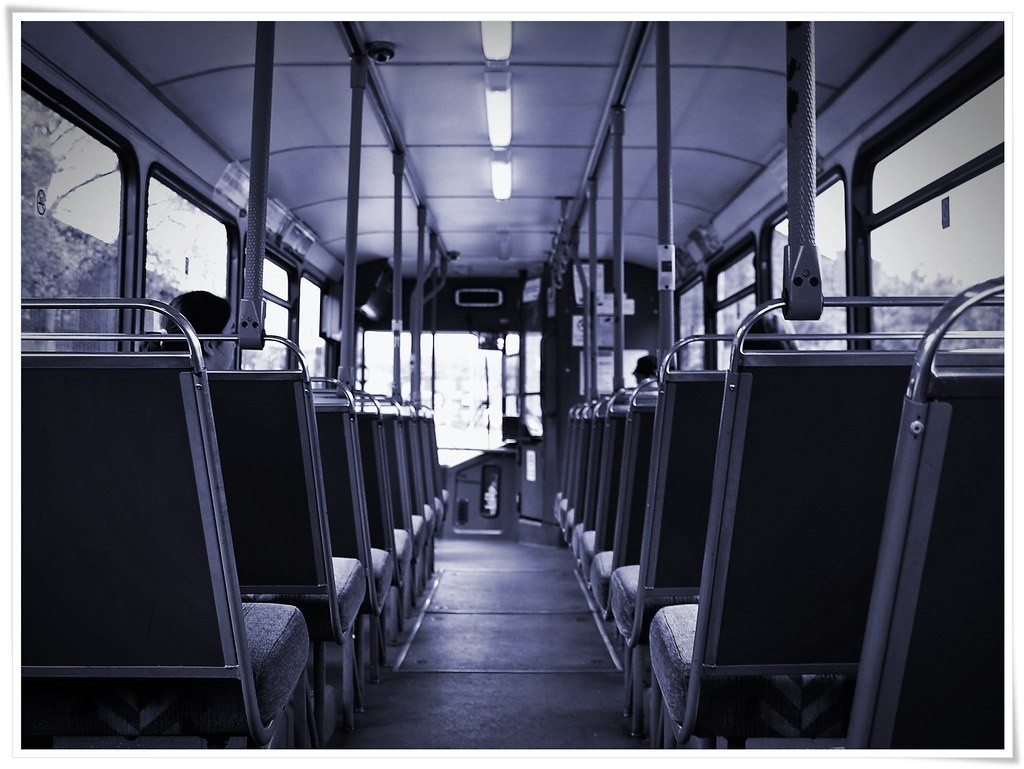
[555,276,1005,748]
[22,295,448,748]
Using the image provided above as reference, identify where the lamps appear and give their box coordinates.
[481,21,512,202]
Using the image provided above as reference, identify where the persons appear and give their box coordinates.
[744,310,799,350]
[146,290,236,370]
[633,355,676,385]
